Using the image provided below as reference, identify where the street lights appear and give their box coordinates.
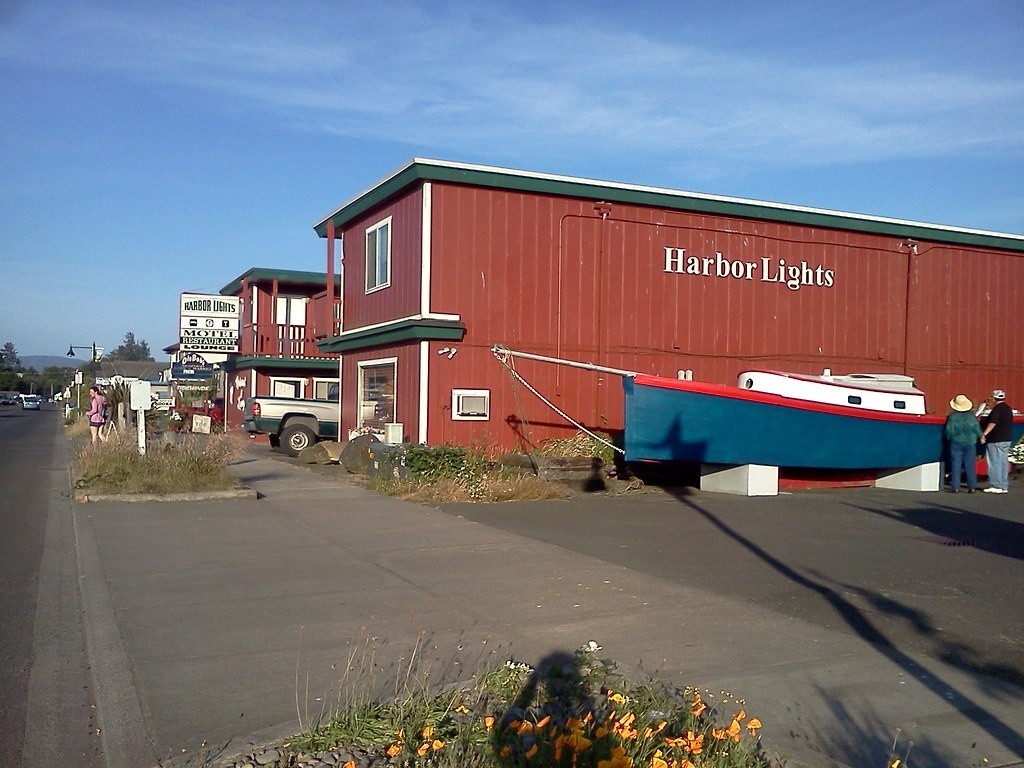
[67,342,97,383]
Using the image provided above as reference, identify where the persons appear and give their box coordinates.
[946,395,985,494]
[981,389,1013,493]
[85,386,106,444]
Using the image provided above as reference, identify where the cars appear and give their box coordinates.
[0,393,23,405]
[22,395,40,411]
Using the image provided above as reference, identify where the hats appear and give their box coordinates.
[992,389,1005,399]
[949,395,973,411]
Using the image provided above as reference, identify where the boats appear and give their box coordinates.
[492,344,1023,477]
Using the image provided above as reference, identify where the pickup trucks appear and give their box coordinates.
[245,381,391,457]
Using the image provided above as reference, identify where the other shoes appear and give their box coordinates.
[1001,490,1008,493]
[969,488,975,493]
[952,488,960,493]
[983,487,1002,493]
